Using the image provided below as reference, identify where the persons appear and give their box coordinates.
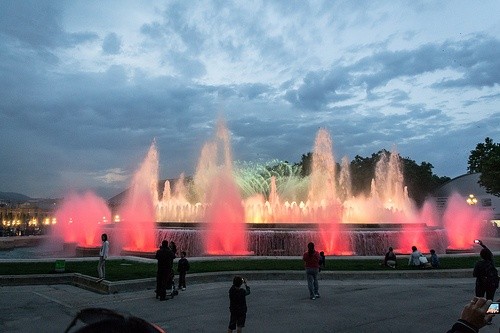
[448,297,494,333]
[430,250,439,266]
[383,246,397,269]
[98,233,109,281]
[408,246,430,269]
[303,242,322,300]
[156,240,191,302]
[228,276,251,333]
[471,240,498,301]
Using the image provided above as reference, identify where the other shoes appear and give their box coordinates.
[95,277,104,283]
[160,297,170,300]
[182,287,186,290]
[178,287,183,290]
[316,293,320,297]
[311,297,316,299]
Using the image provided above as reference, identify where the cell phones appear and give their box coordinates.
[486,302,500,314]
[474,239,479,243]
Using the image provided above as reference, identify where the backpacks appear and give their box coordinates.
[486,262,500,289]
